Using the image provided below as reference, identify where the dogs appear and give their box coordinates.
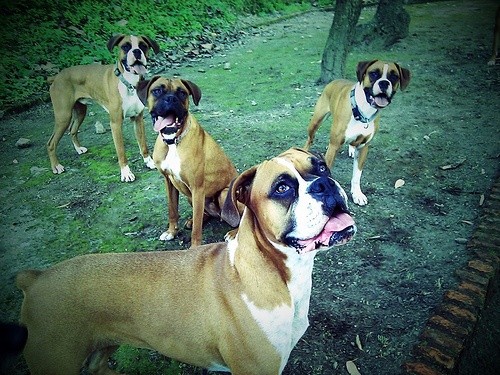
[136,76,245,251]
[17,145,358,375]
[46,33,160,183]
[306,59,412,207]
